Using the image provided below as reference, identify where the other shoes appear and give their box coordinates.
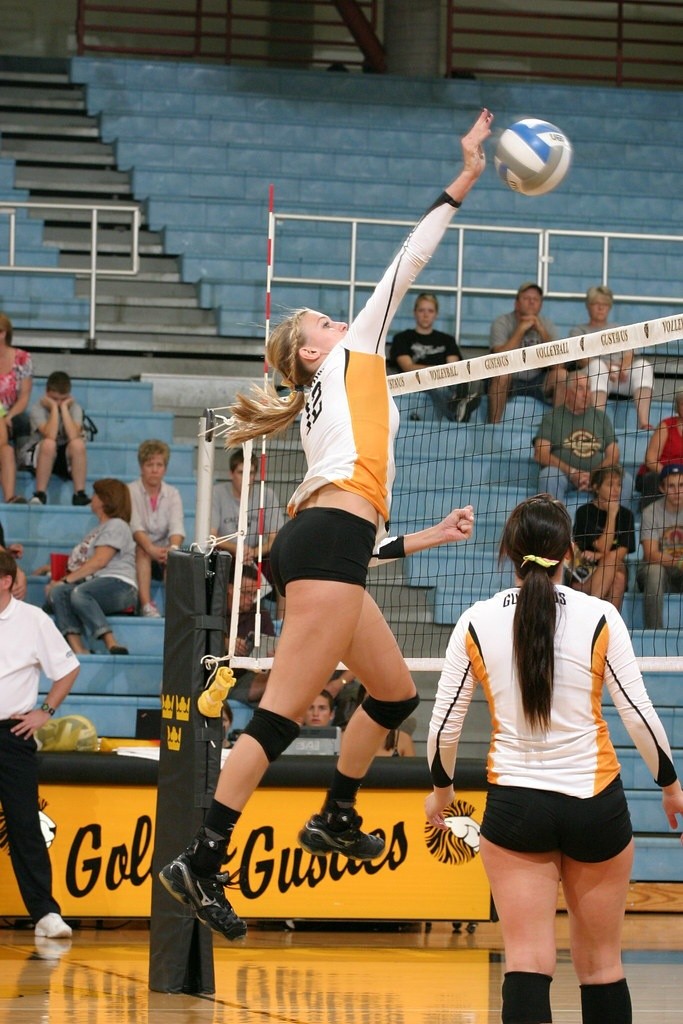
[8,495,27,504]
[109,646,129,655]
[458,392,482,423]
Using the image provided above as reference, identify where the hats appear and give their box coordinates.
[659,463,683,480]
[518,280,542,295]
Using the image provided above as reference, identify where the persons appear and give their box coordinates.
[389,293,481,423]
[158,107,493,944]
[221,563,360,749]
[341,684,415,757]
[423,494,683,1024]
[0,522,27,601]
[210,448,285,637]
[0,312,34,503]
[482,281,569,424]
[126,439,187,617]
[563,466,636,613]
[569,286,655,432]
[0,551,81,937]
[636,464,683,630]
[635,393,683,514]
[33,478,138,655]
[19,371,93,506]
[531,369,641,515]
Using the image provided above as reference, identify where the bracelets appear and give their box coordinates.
[171,543,179,550]
[340,676,347,690]
[62,576,70,584]
[42,703,55,715]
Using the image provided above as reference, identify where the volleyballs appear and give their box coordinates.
[495,118,572,196]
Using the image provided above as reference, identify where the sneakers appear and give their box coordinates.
[158,825,247,942]
[29,492,46,504]
[34,912,72,938]
[73,490,92,505]
[298,789,385,860]
[137,601,162,618]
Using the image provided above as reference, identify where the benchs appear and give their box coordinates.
[1,53,683,883]
